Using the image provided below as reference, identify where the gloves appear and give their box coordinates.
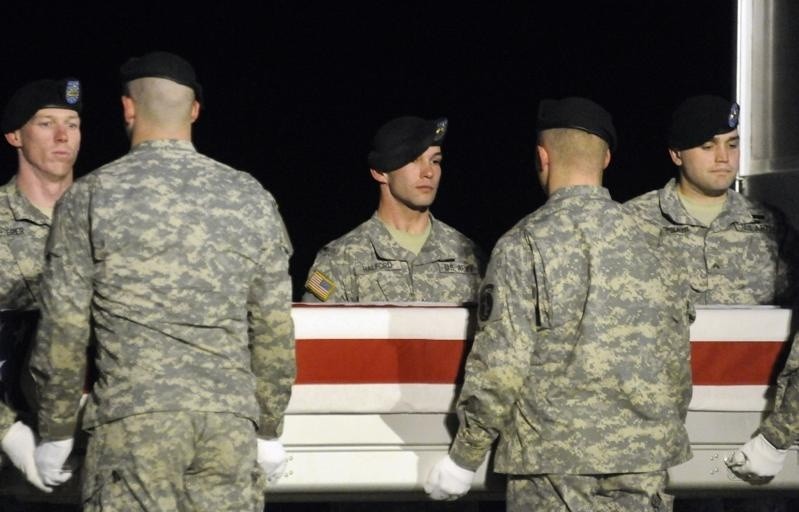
[729,436,790,483]
[425,456,475,500]
[3,419,72,493]
[255,439,289,483]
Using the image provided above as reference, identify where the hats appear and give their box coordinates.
[364,116,448,172]
[2,79,83,133]
[117,52,202,93]
[536,97,620,152]
[664,95,739,149]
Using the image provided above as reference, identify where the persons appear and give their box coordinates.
[624,90,796,309]
[723,319,798,490]
[0,75,110,511]
[303,112,483,306]
[422,97,707,510]
[25,49,296,512]
